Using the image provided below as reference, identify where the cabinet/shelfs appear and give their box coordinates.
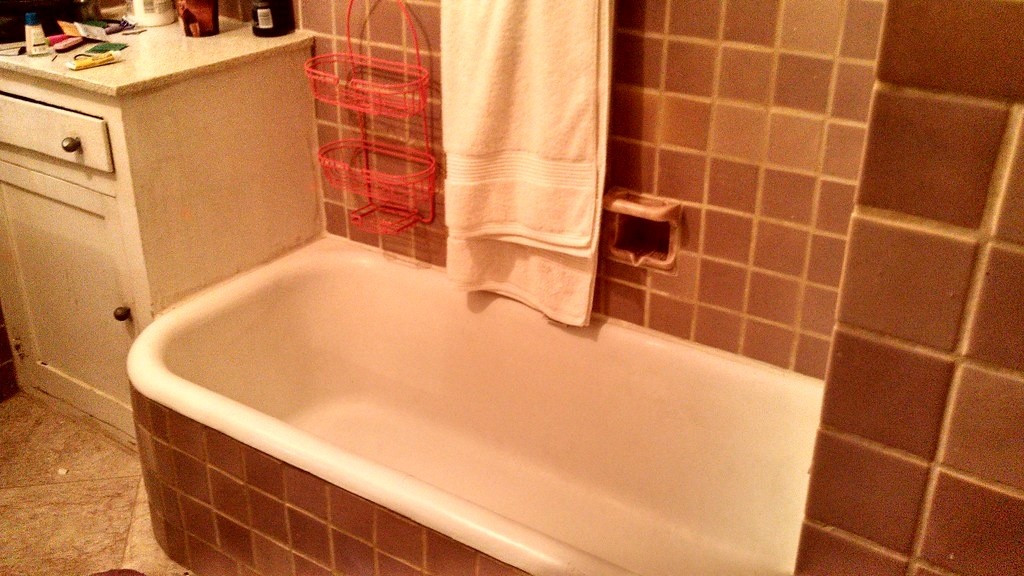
[0,8,327,451]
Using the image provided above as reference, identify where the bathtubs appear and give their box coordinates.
[125,234,825,576]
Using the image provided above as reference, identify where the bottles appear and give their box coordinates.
[251,0,294,37]
[176,0,219,37]
[127,0,177,27]
[25,13,50,57]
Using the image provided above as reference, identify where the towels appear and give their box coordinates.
[439,0,617,328]
